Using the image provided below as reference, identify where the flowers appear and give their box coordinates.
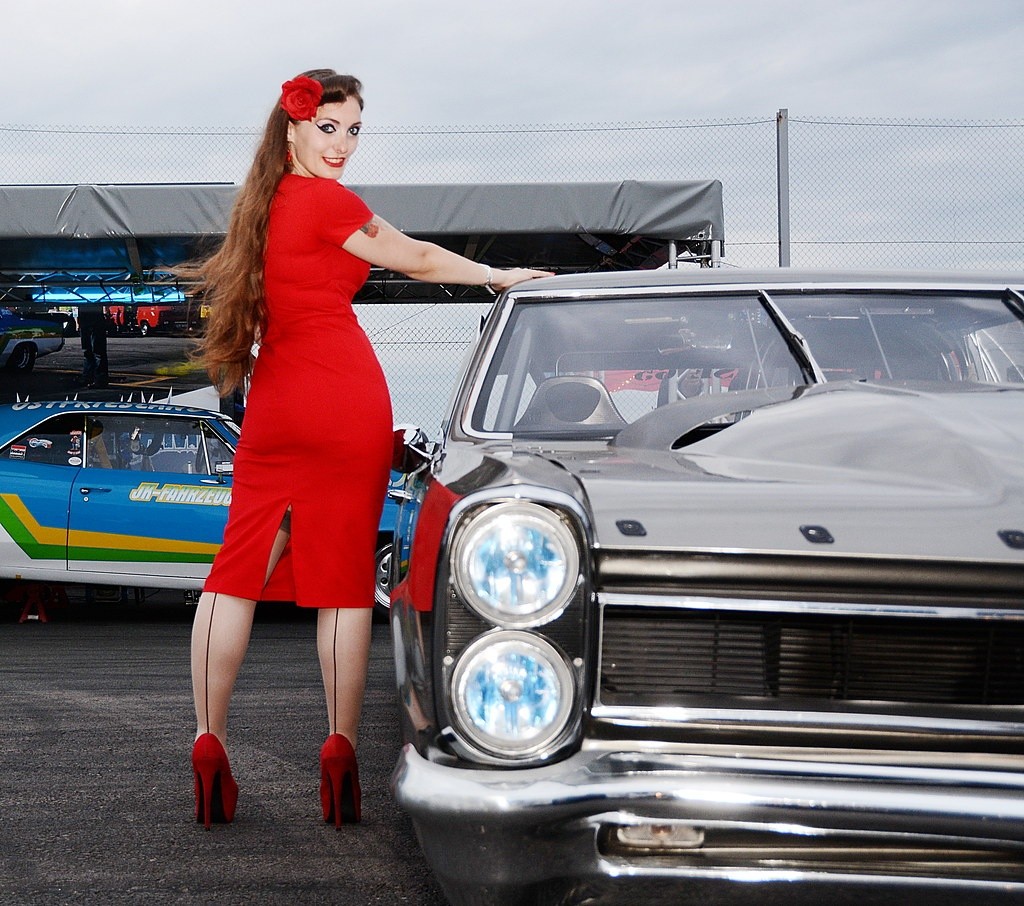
[278,75,324,123]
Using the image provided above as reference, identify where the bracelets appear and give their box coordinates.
[479,264,493,285]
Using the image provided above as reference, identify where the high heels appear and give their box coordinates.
[319,733,361,829]
[192,732,238,829]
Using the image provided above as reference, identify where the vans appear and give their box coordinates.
[101,303,200,338]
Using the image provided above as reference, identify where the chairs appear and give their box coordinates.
[512,375,628,427]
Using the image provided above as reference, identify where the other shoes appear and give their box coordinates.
[87,382,108,389]
[76,380,88,387]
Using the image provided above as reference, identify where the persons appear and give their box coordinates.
[72,306,109,388]
[160,68,555,833]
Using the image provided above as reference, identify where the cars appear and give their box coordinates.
[388,270,1024,906]
[0,308,65,376]
[1,403,422,627]
